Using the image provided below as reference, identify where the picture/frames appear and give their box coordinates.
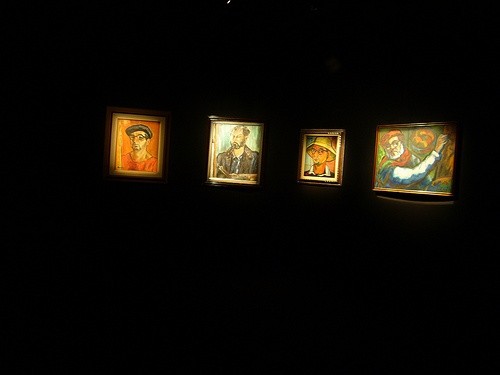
[297,129,345,185]
[204,115,266,190]
[109,112,169,180]
[371,122,459,199]
